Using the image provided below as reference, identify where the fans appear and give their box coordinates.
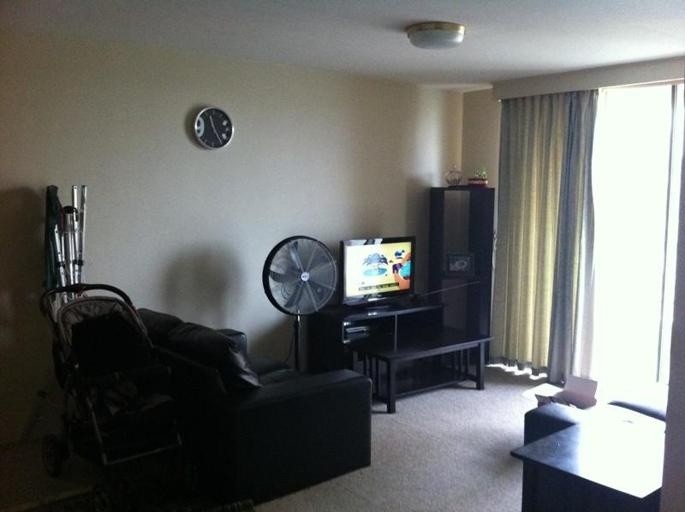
[261,235,339,372]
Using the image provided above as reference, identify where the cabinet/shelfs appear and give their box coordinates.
[429,185,496,365]
[324,304,497,413]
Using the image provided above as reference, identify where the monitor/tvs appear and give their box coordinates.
[339,234,417,310]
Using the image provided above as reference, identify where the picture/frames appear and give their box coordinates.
[444,251,476,276]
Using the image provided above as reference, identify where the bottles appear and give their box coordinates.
[445,163,464,186]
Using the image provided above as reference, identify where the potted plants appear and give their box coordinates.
[466,167,489,186]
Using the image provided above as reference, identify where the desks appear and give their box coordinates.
[511,403,666,512]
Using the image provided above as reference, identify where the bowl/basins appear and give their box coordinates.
[468,178,489,188]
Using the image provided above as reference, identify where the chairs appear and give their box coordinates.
[129,306,374,503]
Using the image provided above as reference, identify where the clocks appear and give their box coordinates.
[406,20,466,50]
[194,106,235,151]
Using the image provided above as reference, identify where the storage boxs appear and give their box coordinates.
[534,375,599,409]
